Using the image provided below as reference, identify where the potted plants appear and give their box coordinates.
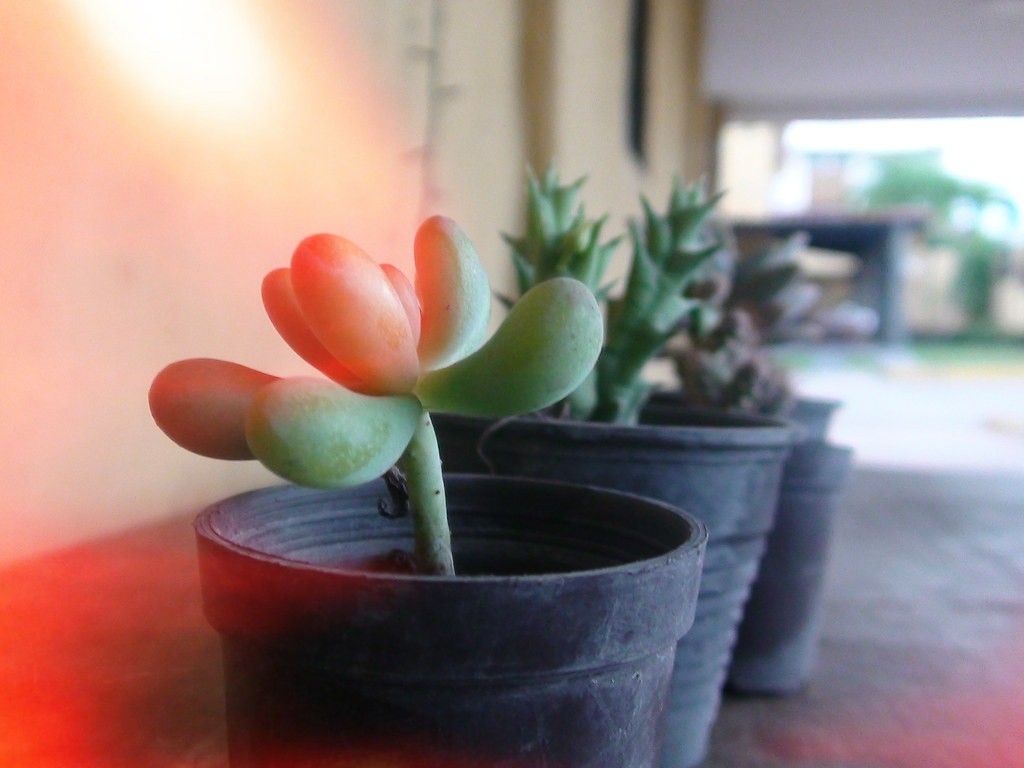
[148,216,708,766]
[425,163,804,766]
[618,209,854,694]
